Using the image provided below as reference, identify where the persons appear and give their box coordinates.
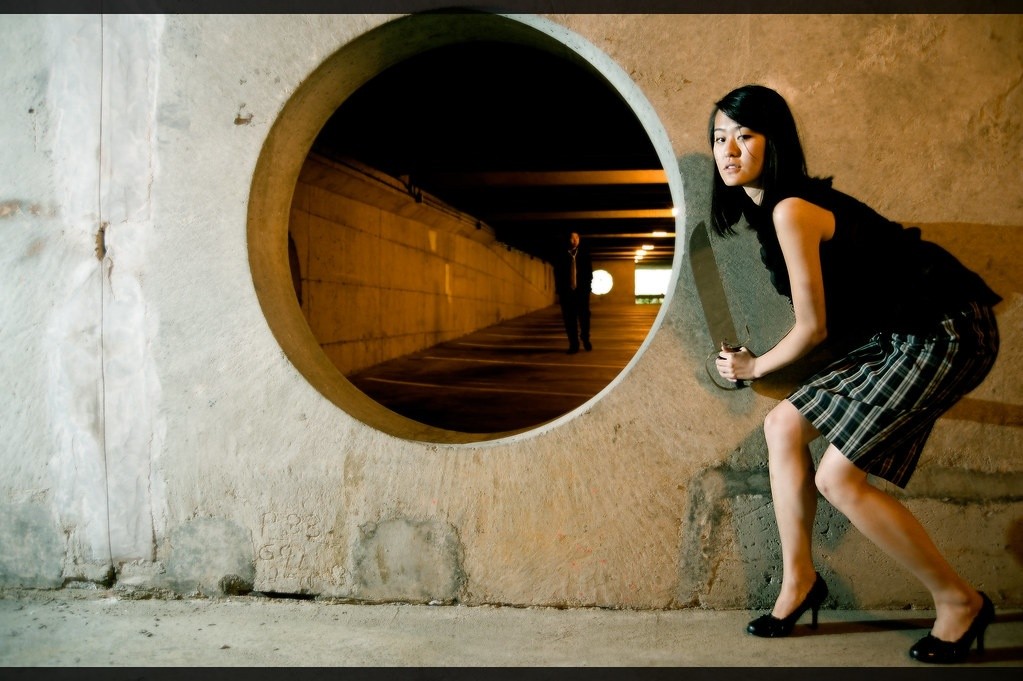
[710,84,1002,661]
[554,231,593,354]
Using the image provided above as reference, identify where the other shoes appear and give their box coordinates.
[581,334,592,351]
[565,341,580,354]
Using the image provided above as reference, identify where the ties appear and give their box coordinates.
[568,248,577,289]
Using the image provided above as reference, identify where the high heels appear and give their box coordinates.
[747,571,828,637]
[908,590,995,664]
